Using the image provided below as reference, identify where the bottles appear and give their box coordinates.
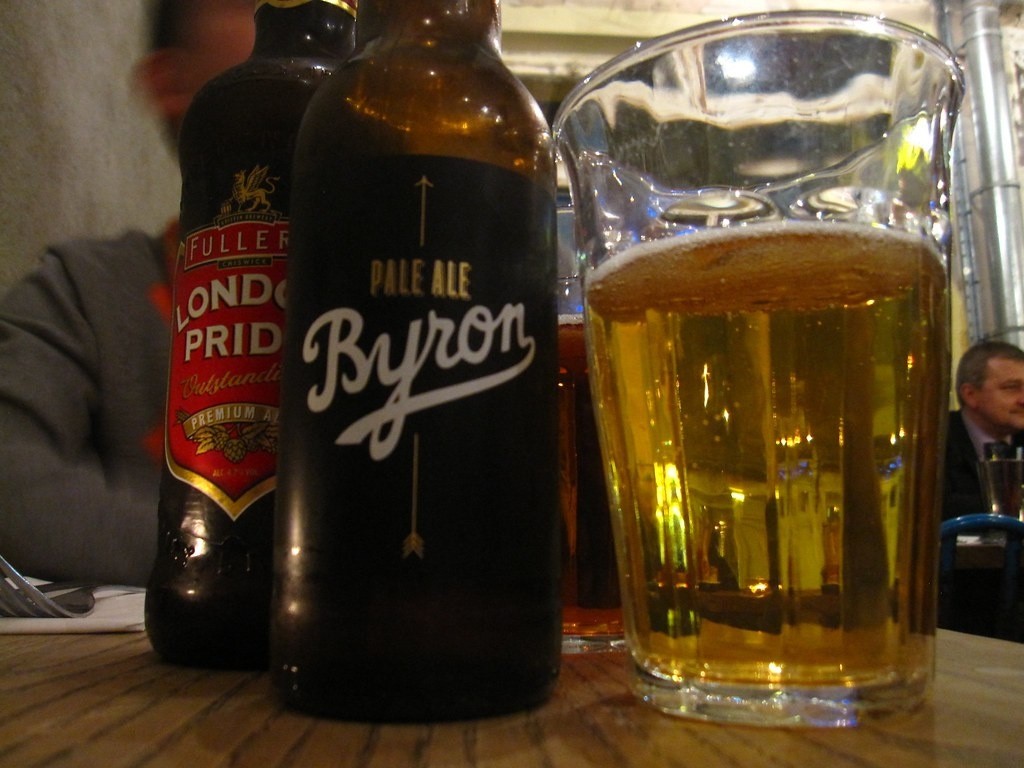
[142,4,350,678]
[272,0,568,731]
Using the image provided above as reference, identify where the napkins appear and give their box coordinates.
[0,575,150,633]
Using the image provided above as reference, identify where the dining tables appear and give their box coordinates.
[1,603,1024,767]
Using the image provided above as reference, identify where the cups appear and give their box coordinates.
[545,4,977,731]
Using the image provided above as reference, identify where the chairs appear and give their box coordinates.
[938,514,1024,642]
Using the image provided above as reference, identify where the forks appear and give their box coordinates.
[0,556,144,619]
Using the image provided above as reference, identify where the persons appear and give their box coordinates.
[0,0,300,592]
[912,339,1023,631]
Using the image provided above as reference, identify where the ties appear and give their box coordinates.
[988,444,1010,460]
